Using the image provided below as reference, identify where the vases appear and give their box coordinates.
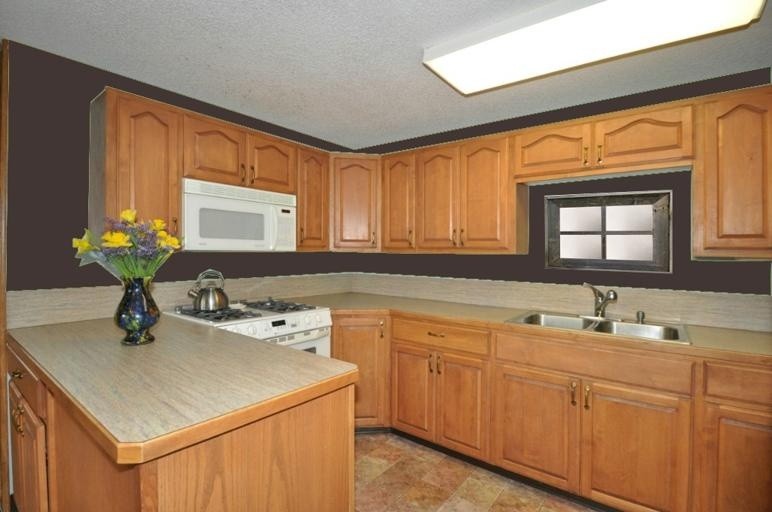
[108,277,161,345]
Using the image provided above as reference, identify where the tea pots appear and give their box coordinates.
[188,269,230,313]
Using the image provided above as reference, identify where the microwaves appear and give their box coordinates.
[180,176,297,252]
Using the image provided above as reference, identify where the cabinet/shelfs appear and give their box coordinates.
[88,87,183,249]
[184,112,296,194]
[332,313,394,429]
[497,324,696,512]
[0,315,363,512]
[340,151,384,254]
[298,145,332,255]
[395,312,492,460]
[414,129,513,258]
[701,353,769,512]
[380,150,418,253]
[688,86,772,256]
[513,98,696,179]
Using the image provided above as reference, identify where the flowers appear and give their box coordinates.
[69,209,182,271]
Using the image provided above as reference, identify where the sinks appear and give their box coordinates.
[511,313,596,343]
[588,316,692,346]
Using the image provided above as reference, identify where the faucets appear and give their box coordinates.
[578,281,618,319]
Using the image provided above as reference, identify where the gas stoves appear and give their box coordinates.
[181,296,334,341]
